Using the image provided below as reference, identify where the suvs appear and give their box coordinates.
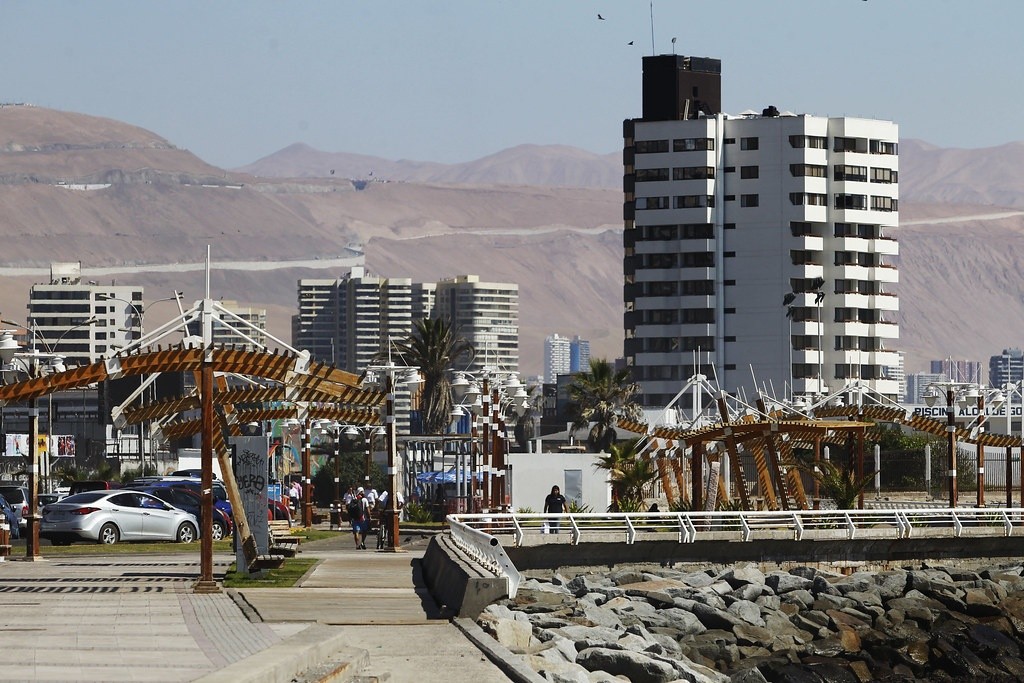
[71,480,123,496]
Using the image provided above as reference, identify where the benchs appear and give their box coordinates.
[241,534,285,571]
[268,530,298,554]
[268,520,306,543]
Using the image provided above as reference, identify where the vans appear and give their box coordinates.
[0,485,31,529]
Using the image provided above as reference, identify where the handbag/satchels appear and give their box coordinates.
[540,522,549,534]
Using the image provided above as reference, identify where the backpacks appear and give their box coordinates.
[349,497,363,518]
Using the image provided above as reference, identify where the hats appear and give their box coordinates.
[357,487,364,492]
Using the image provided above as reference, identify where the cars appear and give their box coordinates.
[36,493,70,513]
[128,476,290,540]
[0,497,21,542]
[40,489,199,545]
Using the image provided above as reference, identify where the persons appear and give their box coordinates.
[284,480,302,515]
[343,481,379,550]
[432,483,448,504]
[648,503,663,525]
[544,485,570,533]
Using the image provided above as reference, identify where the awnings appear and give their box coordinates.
[417,470,457,484]
[449,468,484,483]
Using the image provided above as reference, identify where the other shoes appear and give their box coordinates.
[356,543,367,549]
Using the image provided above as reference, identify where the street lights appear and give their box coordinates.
[781,276,827,399]
[451,369,529,536]
[2,318,98,493]
[100,294,187,477]
[920,380,1020,507]
[364,364,427,550]
